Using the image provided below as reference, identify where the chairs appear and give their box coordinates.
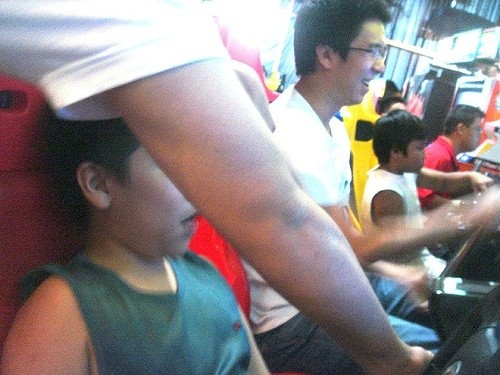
[0,73,379,375]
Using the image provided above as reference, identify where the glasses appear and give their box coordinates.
[334,46,390,59]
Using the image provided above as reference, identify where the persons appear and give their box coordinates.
[1,0,435,375]
[361,110,496,291]
[240,0,500,375]
[372,79,486,204]
[0,97,271,375]
